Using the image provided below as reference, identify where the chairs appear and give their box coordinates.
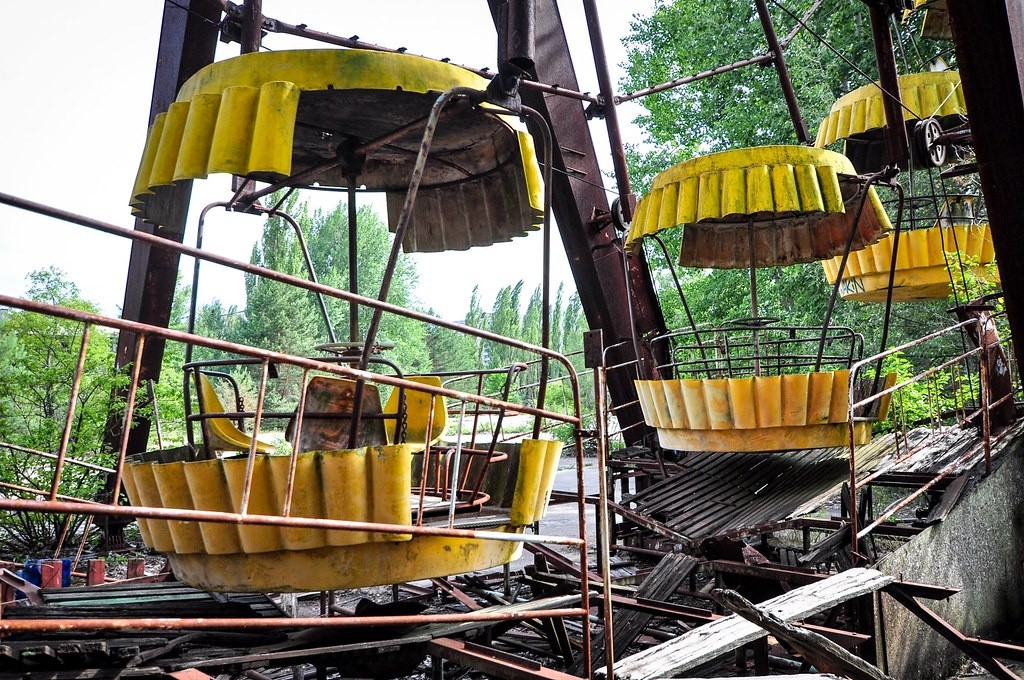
[190,371,278,455]
[285,378,389,453]
[384,373,450,454]
[933,196,976,227]
